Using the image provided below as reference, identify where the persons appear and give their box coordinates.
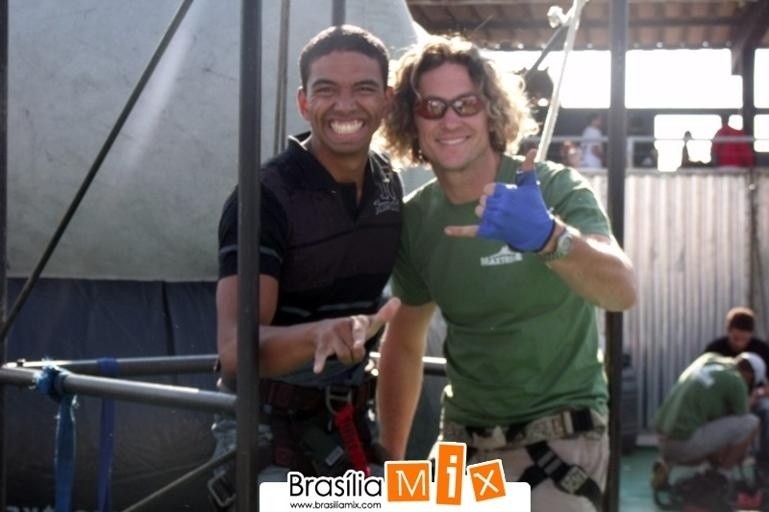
[651,307,769,502]
[375,35,639,512]
[211,25,403,512]
[711,115,755,167]
[579,113,606,168]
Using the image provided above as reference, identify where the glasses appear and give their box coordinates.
[413,93,487,120]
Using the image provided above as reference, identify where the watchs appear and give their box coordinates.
[538,225,573,262]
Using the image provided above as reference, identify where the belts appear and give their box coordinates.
[439,412,596,449]
[213,359,377,414]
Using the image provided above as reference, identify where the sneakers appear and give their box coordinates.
[651,462,669,490]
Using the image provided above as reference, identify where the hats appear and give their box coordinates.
[741,352,767,395]
